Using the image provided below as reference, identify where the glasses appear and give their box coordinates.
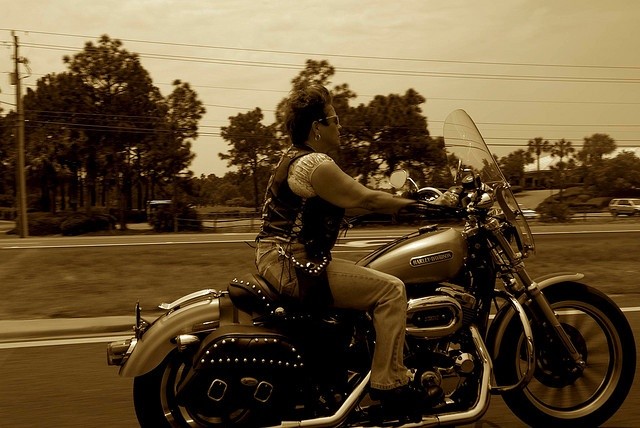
[312,114,340,126]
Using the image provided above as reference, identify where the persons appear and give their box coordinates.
[255,84,460,403]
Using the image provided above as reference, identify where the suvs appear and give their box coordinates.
[609,198,640,216]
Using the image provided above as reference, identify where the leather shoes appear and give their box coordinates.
[370,383,445,409]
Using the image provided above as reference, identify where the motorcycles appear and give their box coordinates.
[108,110,636,428]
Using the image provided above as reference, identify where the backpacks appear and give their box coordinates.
[173,296,303,417]
